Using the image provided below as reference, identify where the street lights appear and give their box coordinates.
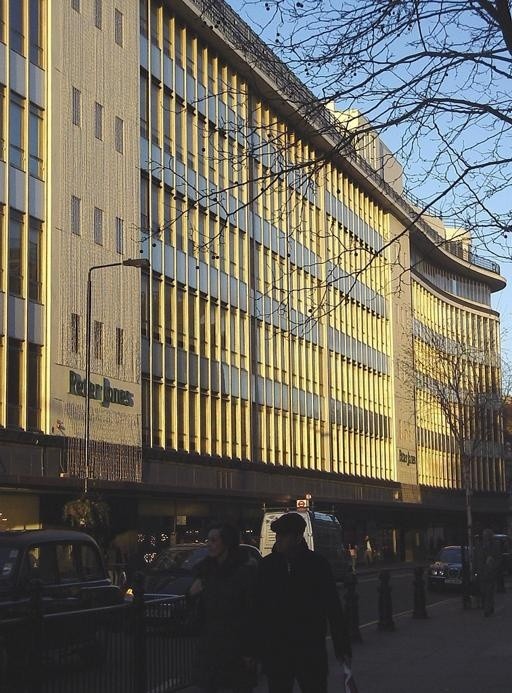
[85,258,151,499]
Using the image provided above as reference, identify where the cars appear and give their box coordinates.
[119,542,262,634]
[0,529,122,670]
[427,533,512,590]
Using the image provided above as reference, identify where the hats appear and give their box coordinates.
[270,513,307,532]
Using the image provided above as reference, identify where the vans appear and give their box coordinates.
[260,502,347,581]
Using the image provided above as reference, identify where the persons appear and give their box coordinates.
[238,510,355,692]
[361,533,376,563]
[182,521,273,691]
[473,527,500,618]
